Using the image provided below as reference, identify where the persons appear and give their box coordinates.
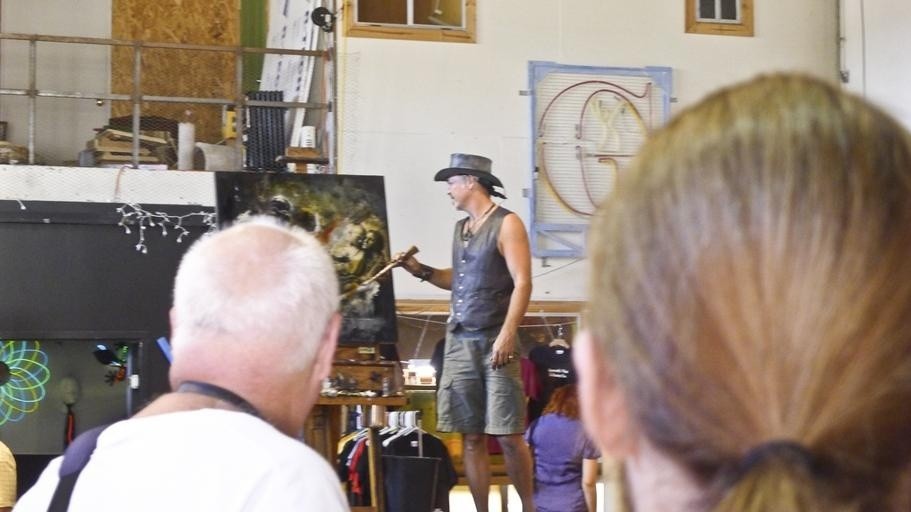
[8,209,357,511]
[337,230,388,308]
[330,223,367,302]
[389,151,536,512]
[522,380,603,512]
[563,73,911,511]
[1,359,20,512]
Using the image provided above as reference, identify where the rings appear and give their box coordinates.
[509,355,514,358]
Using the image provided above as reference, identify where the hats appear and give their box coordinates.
[435,154,503,188]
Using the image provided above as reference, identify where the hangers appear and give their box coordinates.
[528,325,580,363]
[336,409,448,458]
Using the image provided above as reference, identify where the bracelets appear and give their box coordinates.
[412,264,435,281]
